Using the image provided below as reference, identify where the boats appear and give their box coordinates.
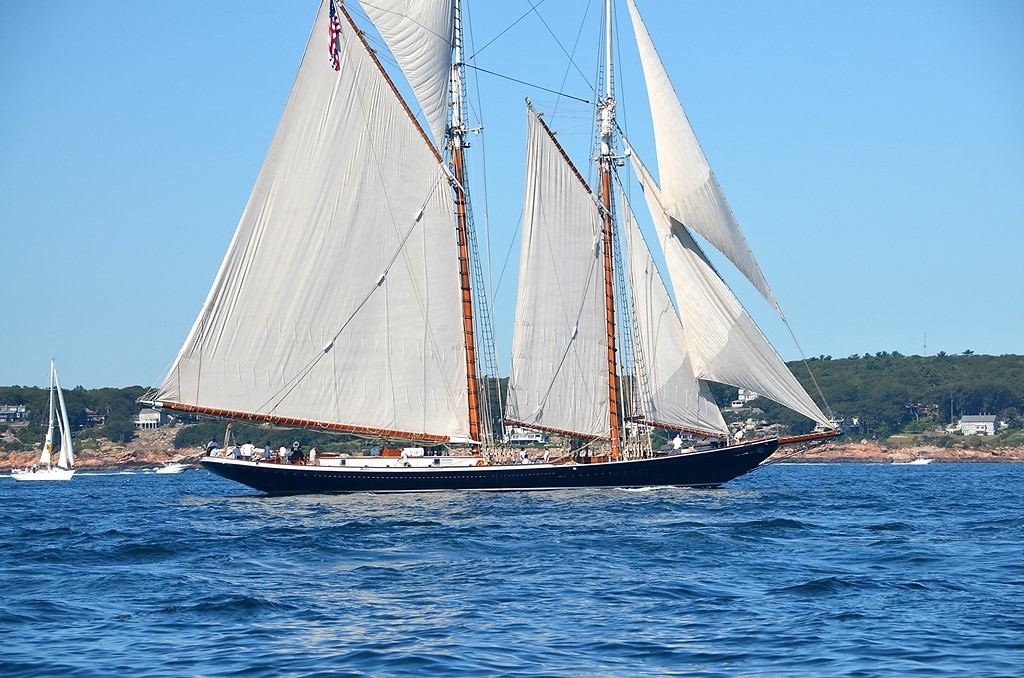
[910,457,935,465]
[152,463,192,474]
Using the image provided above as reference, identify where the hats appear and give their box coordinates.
[211,437,216,440]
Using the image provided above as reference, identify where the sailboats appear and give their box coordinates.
[7,357,77,481]
[128,0,848,500]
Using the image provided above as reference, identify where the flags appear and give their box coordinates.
[328,0,342,71]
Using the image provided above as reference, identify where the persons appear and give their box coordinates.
[542,447,550,462]
[228,444,242,459]
[264,441,271,459]
[522,455,532,464]
[309,445,317,462]
[240,440,255,461]
[399,447,412,467]
[710,438,718,448]
[207,438,218,455]
[672,433,682,454]
[279,442,304,464]
[520,447,525,461]
[734,429,746,442]
[210,447,221,457]
